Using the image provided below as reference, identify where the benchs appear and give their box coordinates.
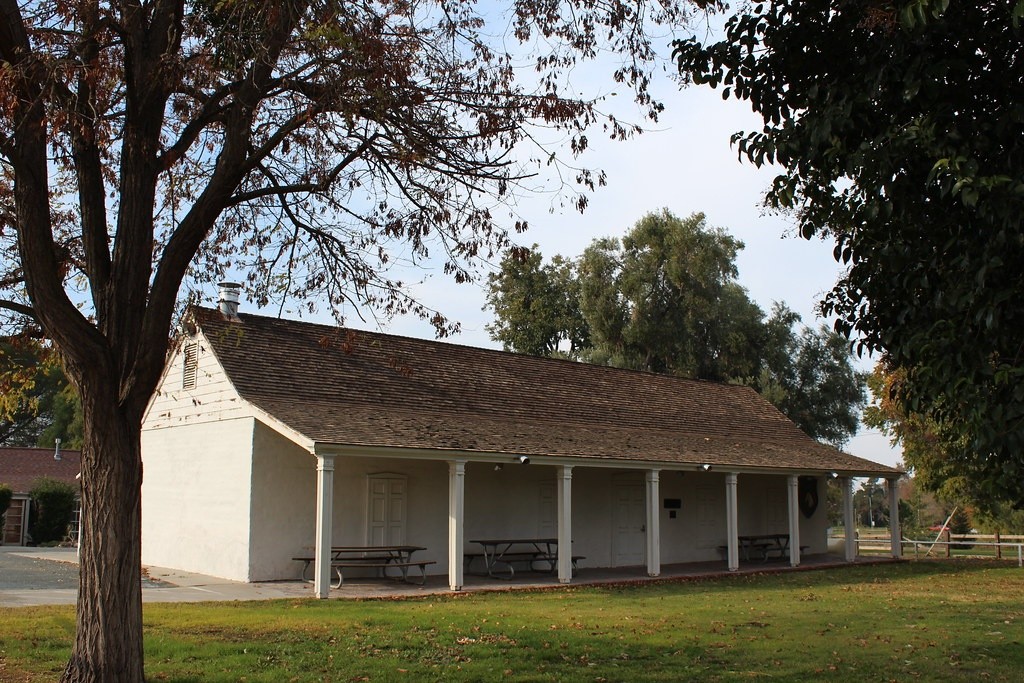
[464,552,551,576]
[291,556,408,584]
[330,560,437,589]
[493,556,587,581]
[750,545,810,565]
[718,543,773,561]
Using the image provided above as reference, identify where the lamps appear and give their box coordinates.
[832,473,838,478]
[702,463,712,471]
[518,455,530,464]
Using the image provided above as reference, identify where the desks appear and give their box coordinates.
[469,538,574,576]
[738,533,789,562]
[314,545,428,581]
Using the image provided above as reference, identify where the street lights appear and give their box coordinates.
[863,495,874,528]
